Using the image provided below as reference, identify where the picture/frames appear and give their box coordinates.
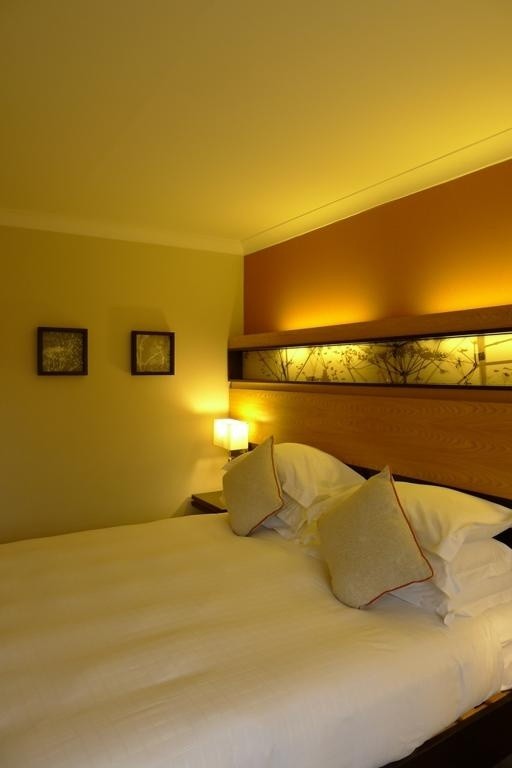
[35,325,88,376]
[131,329,176,377]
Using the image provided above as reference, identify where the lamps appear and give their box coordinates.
[211,414,253,462]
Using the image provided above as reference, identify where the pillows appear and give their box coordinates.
[260,514,300,540]
[313,479,512,558]
[425,537,511,598]
[228,443,365,505]
[277,490,333,532]
[301,527,512,622]
[318,463,431,609]
[224,437,286,535]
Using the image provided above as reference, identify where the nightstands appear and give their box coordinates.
[190,490,229,514]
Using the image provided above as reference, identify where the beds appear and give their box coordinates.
[0,463,512,767]
